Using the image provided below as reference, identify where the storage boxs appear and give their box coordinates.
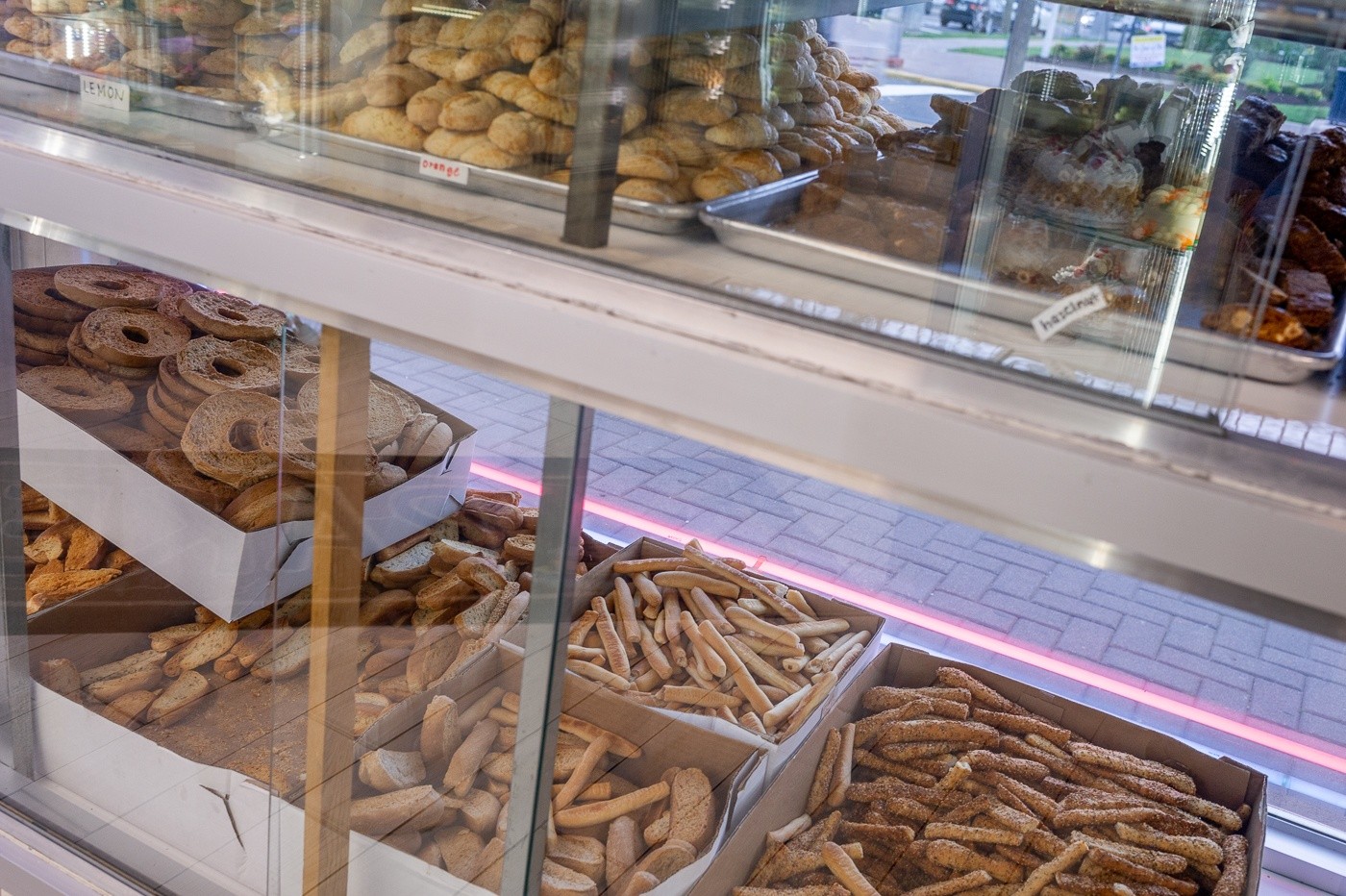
[0,264,1271,896]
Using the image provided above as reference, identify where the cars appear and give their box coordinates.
[939,0,1052,37]
[1081,9,1187,48]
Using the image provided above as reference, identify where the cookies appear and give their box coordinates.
[450,539,872,830]
[731,666,1256,894]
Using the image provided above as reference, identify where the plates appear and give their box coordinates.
[1022,197,1130,228]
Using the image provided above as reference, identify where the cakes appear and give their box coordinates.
[1021,134,1142,217]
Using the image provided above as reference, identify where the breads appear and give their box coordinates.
[343,695,720,896]
[0,0,906,213]
[14,475,143,620]
[773,70,1346,360]
[9,260,453,531]
[35,489,588,732]
